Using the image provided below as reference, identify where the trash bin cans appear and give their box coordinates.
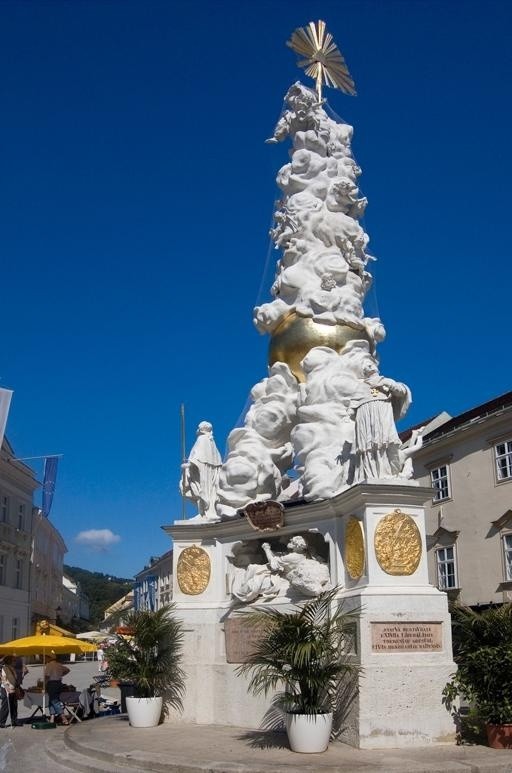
[121,685,137,713]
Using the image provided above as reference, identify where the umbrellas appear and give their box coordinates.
[75,629,110,661]
[0,632,98,720]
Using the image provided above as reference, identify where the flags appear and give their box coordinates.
[42,456,57,517]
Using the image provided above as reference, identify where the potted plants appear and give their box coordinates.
[234,582,368,754]
[94,600,188,728]
[442,644,512,749]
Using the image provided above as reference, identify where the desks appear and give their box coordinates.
[22,690,81,723]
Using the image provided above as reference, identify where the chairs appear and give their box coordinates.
[65,688,92,724]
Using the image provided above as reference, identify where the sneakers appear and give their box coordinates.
[0,723,11,728]
[12,722,23,726]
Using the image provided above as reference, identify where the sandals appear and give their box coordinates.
[62,720,69,725]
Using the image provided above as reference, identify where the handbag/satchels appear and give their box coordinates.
[15,686,25,700]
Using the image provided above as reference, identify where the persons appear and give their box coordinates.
[1,655,24,728]
[173,419,222,515]
[218,79,425,508]
[97,643,107,671]
[231,532,307,605]
[45,653,70,728]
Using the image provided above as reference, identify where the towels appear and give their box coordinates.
[79,689,92,717]
